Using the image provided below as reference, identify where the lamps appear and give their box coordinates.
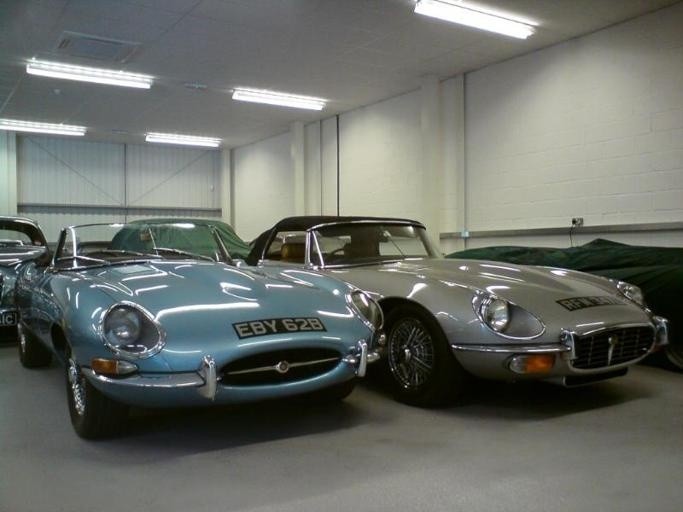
[140,128,225,152]
[411,0,536,42]
[225,84,329,114]
[0,117,89,139]
[20,57,155,95]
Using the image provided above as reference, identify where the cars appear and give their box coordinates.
[646,283,682,375]
[0,213,53,335]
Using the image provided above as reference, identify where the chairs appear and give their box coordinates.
[277,239,311,266]
[340,239,381,260]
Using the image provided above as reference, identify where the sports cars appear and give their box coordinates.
[249,212,670,414]
[11,217,387,450]
